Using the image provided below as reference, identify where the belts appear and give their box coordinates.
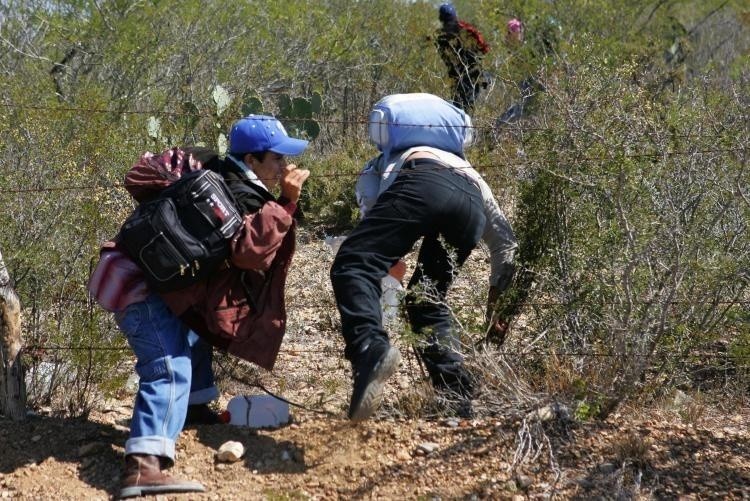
[402,158,479,188]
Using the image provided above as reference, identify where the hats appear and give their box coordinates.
[439,4,456,20]
[508,19,522,33]
[229,114,310,156]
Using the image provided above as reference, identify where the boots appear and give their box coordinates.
[120,455,207,500]
[184,405,231,425]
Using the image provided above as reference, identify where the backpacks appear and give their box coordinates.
[114,169,264,294]
[369,92,473,172]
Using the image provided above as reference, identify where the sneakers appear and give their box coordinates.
[348,340,399,418]
[422,399,471,418]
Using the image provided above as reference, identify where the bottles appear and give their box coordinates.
[218,393,290,428]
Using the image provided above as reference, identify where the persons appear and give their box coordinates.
[330,146,518,423]
[87,114,310,498]
[505,19,525,41]
[433,4,489,113]
